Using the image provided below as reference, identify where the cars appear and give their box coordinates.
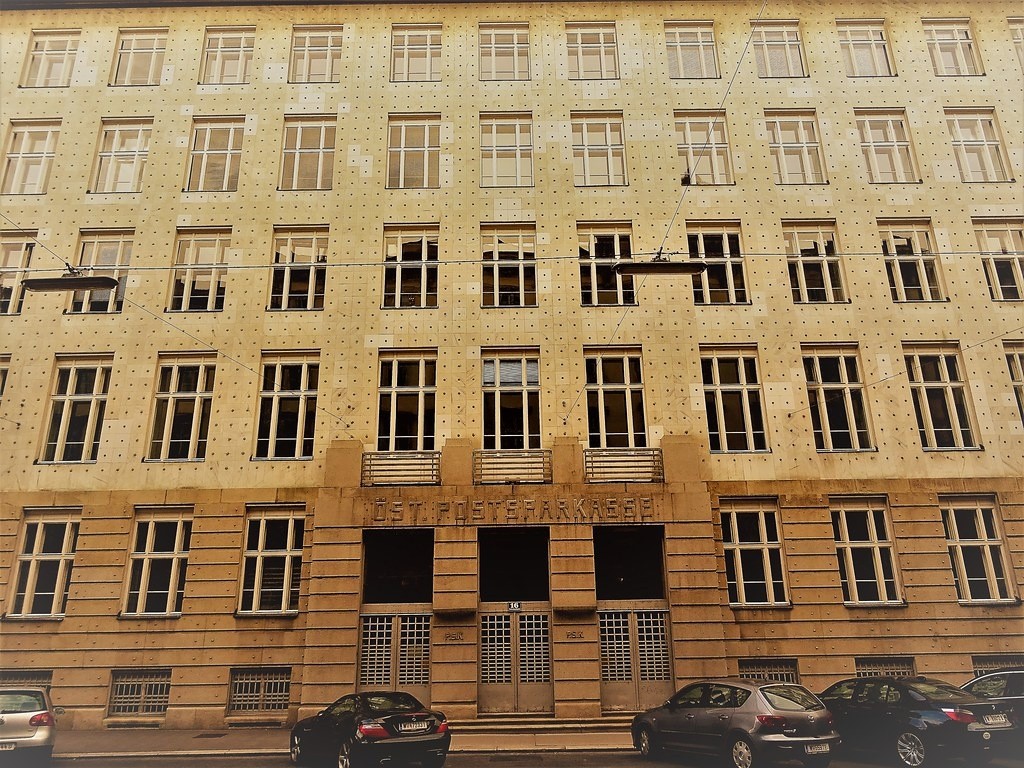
[0,684,65,767]
[630,677,841,768]
[290,691,452,768]
[958,665,1024,757]
[819,676,1020,768]
